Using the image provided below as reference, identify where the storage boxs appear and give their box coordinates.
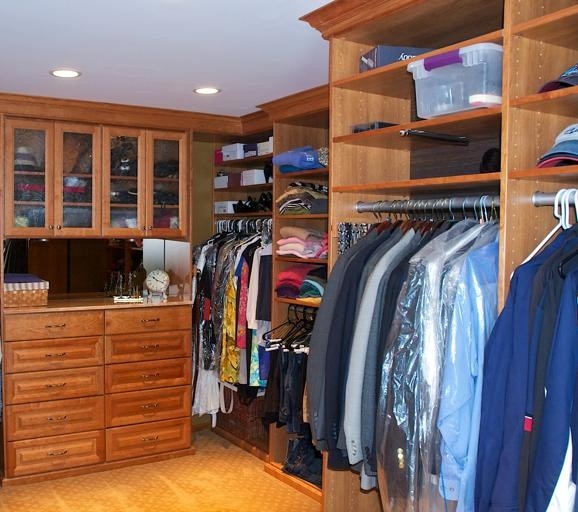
[356,46,437,75]
[209,133,275,216]
[404,41,503,120]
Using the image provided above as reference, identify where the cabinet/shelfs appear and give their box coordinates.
[297,1,512,511]
[494,1,577,318]
[106,294,199,474]
[253,81,333,503]
[197,94,277,467]
[1,92,104,240]
[2,292,106,487]
[100,101,193,244]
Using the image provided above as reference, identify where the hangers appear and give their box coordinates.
[208,219,273,244]
[507,185,578,281]
[368,193,498,235]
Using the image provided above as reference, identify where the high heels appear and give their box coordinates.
[264,164,272,184]
[232,196,256,213]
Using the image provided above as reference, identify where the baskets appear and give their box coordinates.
[4,277,50,308]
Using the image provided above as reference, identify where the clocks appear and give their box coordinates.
[145,267,171,294]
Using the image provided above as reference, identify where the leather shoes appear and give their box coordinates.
[258,191,272,211]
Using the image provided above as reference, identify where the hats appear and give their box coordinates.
[538,63,578,92]
[536,124,578,167]
